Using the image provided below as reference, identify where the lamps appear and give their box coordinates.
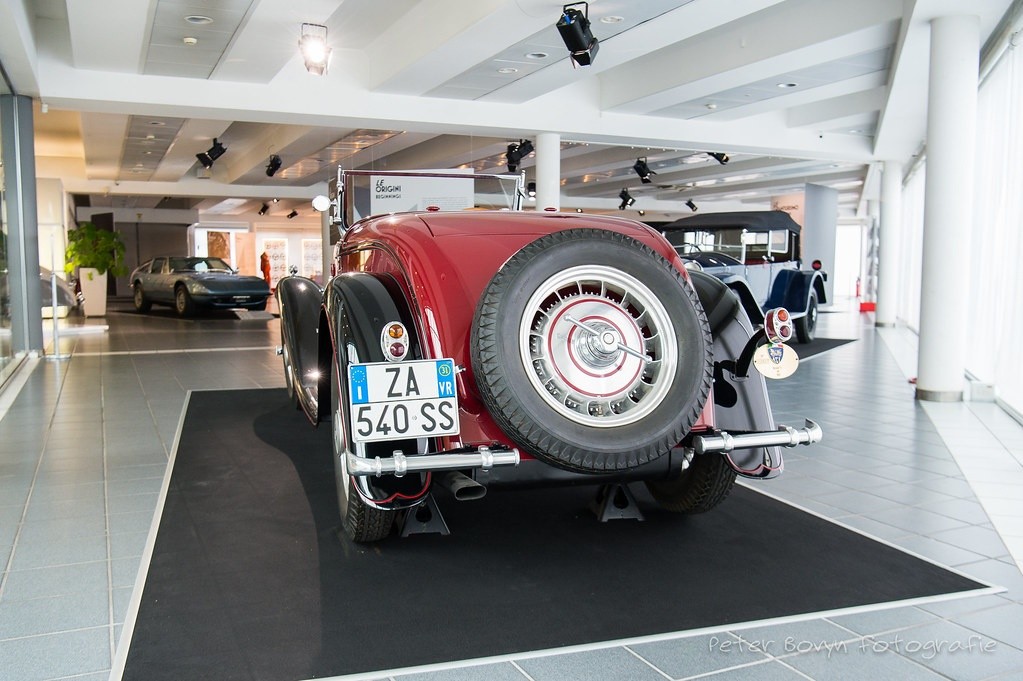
[299,23,333,76]
[708,152,730,165]
[528,182,536,196]
[685,200,698,212]
[506,139,534,172]
[257,198,298,219]
[195,137,227,168]
[618,187,636,210]
[633,157,659,183]
[555,1,599,68]
[265,154,281,176]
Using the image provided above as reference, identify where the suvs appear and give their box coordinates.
[272,163,822,542]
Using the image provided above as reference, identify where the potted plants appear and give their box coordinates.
[66,221,128,318]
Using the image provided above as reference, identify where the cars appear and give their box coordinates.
[660,211,827,343]
[127,256,273,317]
